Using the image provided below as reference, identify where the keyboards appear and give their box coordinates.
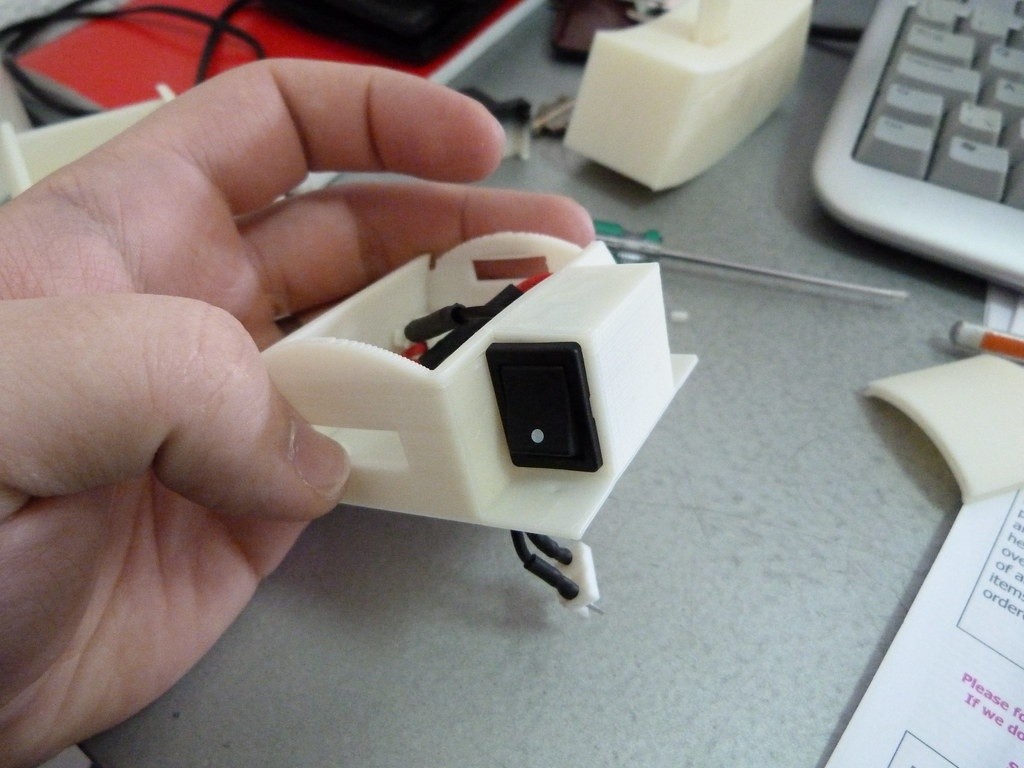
[810,0,1024,292]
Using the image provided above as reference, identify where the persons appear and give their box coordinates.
[0,58,594,768]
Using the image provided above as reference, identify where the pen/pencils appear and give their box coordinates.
[950,320,1024,361]
